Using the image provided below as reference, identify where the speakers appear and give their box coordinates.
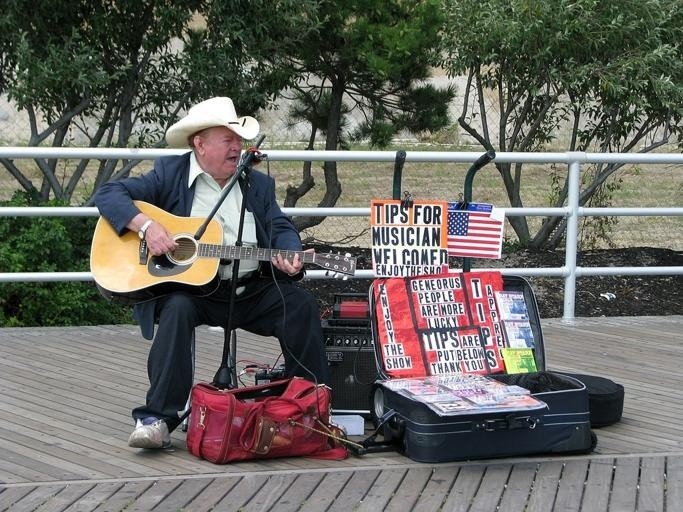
[320,318,382,422]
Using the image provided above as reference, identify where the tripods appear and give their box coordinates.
[169,134,266,433]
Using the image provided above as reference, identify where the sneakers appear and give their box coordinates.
[128,415,173,449]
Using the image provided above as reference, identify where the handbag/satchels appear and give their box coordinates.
[185,378,348,465]
[554,372,626,429]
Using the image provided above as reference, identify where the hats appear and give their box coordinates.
[165,96,260,148]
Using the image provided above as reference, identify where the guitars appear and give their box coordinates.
[90,199,356,304]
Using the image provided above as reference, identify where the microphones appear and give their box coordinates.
[244,146,263,167]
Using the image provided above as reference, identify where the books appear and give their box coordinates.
[494,289,540,374]
[384,376,541,415]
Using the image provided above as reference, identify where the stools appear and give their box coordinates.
[170,319,237,432]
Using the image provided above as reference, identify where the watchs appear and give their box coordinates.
[138,219,152,240]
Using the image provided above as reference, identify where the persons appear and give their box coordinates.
[92,96,330,449]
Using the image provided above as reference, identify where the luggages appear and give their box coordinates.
[368,273,597,464]
